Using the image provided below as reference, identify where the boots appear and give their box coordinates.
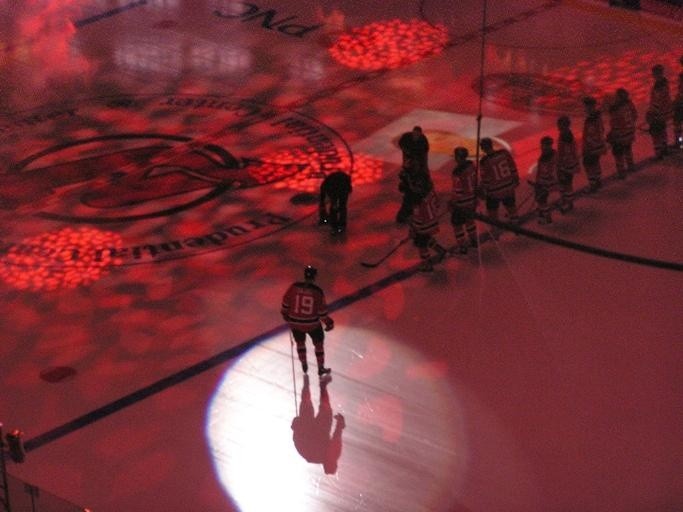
[418,151,670,273]
[302,363,306,372]
[318,366,333,373]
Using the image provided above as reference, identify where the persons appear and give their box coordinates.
[646,64,683,160]
[607,88,637,181]
[450,146,479,257]
[316,169,351,235]
[280,265,333,376]
[396,126,451,272]
[478,137,519,241]
[534,114,580,228]
[582,96,606,198]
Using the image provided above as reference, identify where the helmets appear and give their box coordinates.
[304,265,316,280]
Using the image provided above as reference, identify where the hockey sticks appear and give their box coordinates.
[288,321,296,346]
[505,184,535,218]
[360,235,410,268]
